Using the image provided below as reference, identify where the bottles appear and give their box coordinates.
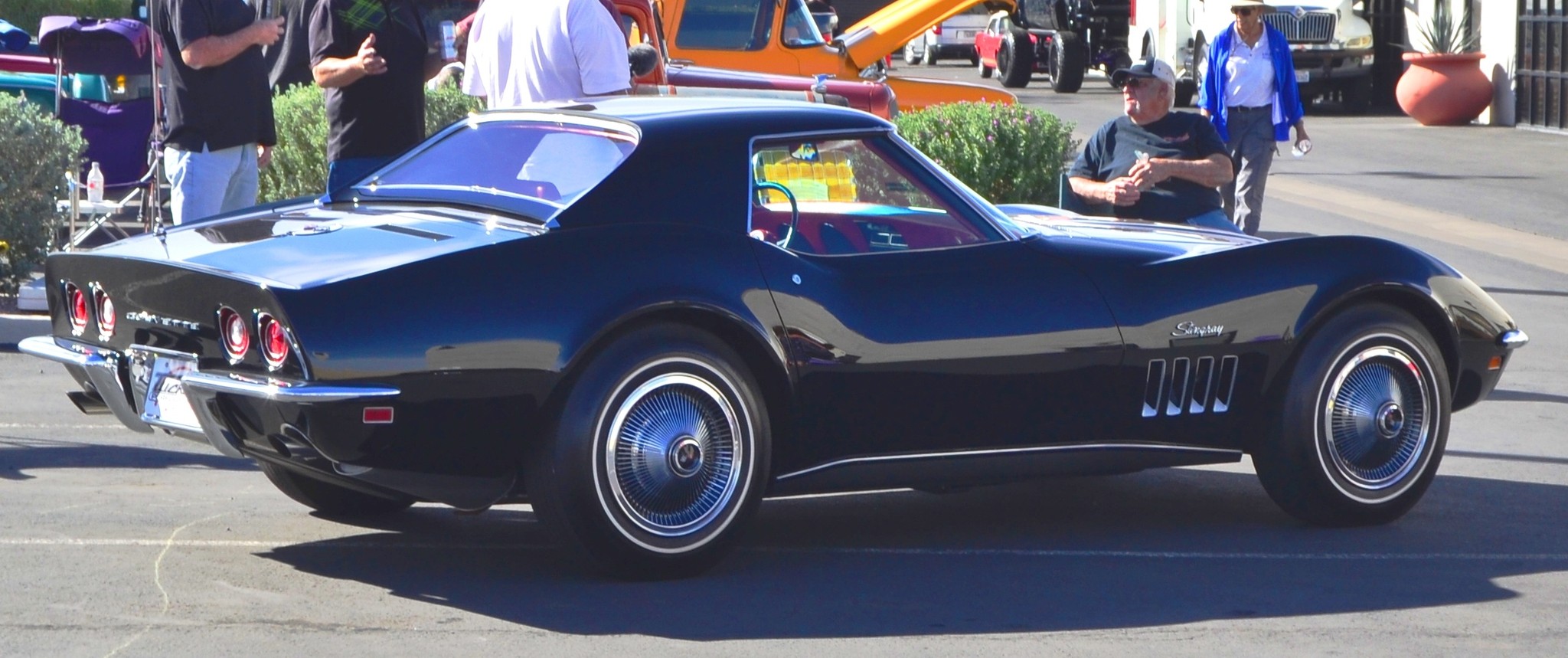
[87,162,105,203]
[1291,139,1312,157]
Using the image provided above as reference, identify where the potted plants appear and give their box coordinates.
[1394,6,1493,126]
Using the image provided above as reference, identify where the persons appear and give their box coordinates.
[462,0,632,110]
[1196,0,1312,236]
[156,0,286,225]
[1067,57,1248,234]
[308,0,466,195]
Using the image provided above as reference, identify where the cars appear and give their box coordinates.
[426,0,1376,202]
[0,17,156,109]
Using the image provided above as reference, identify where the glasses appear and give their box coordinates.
[1231,7,1257,17]
[1118,77,1156,90]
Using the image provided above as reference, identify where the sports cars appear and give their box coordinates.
[16,94,1530,588]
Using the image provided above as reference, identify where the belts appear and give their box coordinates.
[1227,104,1273,111]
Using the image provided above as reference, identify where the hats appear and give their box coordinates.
[1112,57,1176,89]
[1227,0,1275,14]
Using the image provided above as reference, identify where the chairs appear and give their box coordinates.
[38,16,162,243]
